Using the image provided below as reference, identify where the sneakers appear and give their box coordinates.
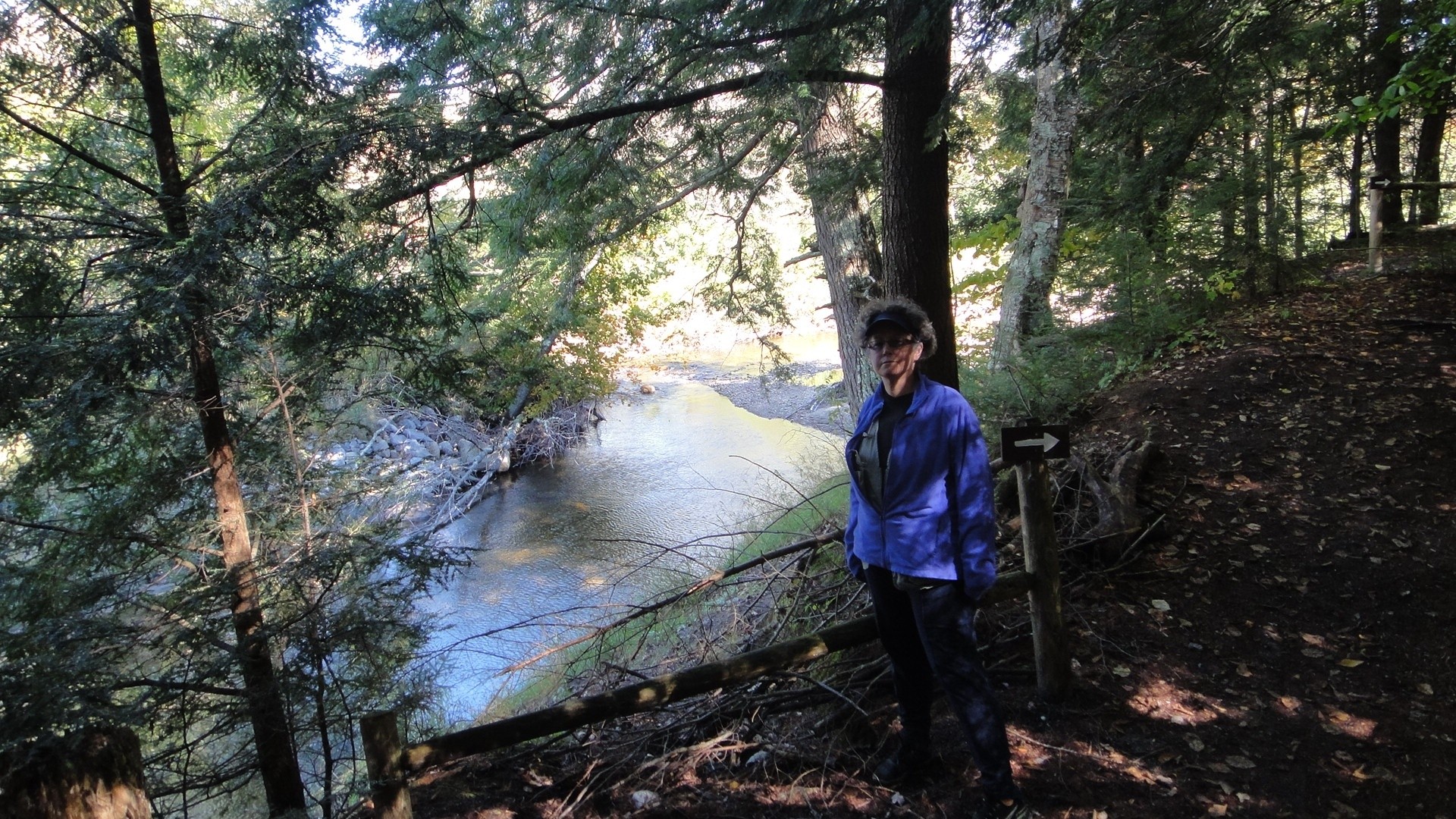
[871,737,935,790]
[969,795,1029,819]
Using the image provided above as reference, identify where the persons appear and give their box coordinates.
[844,303,1024,819]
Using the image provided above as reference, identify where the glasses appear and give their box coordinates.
[867,334,919,351]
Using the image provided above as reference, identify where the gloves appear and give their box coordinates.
[944,581,972,611]
